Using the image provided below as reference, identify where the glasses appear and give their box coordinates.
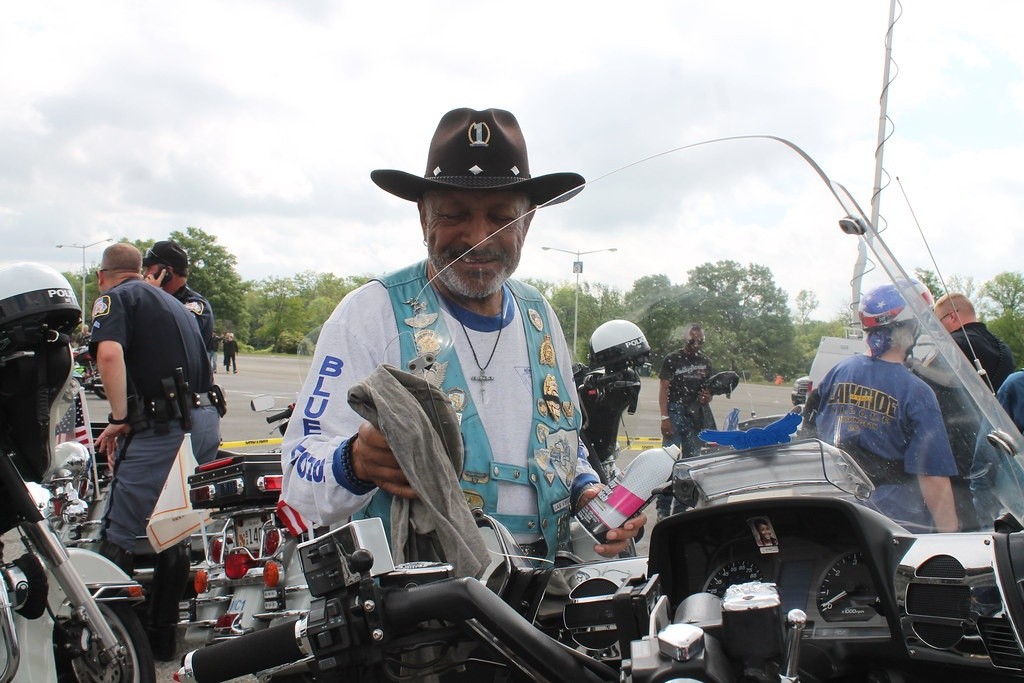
[687,338,705,346]
[96,269,108,279]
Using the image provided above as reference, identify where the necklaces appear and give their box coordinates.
[432,279,505,403]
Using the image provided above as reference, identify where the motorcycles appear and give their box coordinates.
[0,323,1024,683]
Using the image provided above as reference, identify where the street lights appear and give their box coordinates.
[55,238,114,333]
[542,245,619,354]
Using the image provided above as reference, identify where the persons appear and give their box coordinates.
[656,327,713,522]
[815,279,961,534]
[210,331,238,374]
[970,368,1024,531]
[282,108,648,574]
[94,242,227,575]
[71,325,93,378]
[921,294,1014,532]
[144,242,214,354]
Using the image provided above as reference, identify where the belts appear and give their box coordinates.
[191,393,212,406]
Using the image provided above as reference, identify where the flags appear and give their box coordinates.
[55,392,90,449]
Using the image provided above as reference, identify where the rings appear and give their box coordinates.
[666,432,669,434]
[104,437,109,439]
[703,396,704,398]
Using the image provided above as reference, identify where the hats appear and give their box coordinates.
[143,241,188,268]
[370,107,586,207]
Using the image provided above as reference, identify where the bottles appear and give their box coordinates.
[574,445,683,546]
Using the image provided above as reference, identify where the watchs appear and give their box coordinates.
[660,416,669,420]
[108,412,129,425]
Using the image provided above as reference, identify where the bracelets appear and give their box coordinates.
[341,434,376,489]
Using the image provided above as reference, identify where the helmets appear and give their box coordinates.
[0,261,82,348]
[859,278,935,330]
[588,320,651,371]
